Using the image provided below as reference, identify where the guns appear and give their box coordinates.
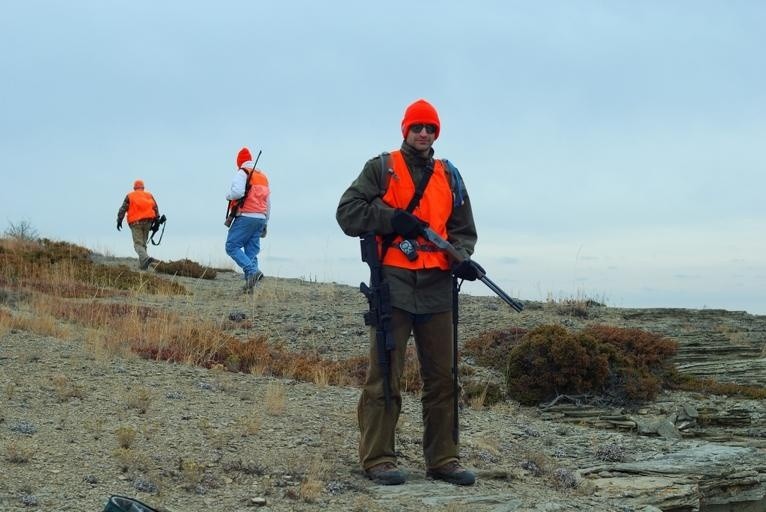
[360,235,395,414]
[372,197,522,313]
[224,150,261,227]
[146,215,165,244]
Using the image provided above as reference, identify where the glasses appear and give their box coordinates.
[410,124,436,134]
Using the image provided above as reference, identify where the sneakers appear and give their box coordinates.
[140,256,155,271]
[426,460,476,486]
[240,270,264,294]
[364,461,406,484]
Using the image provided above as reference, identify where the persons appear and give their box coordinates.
[335,98,478,486]
[225,146,270,294]
[116,178,160,271]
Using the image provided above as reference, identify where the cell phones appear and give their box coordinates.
[399,236,419,262]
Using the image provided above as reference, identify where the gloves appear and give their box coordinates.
[260,225,267,238]
[116,223,122,231]
[155,218,160,226]
[449,256,477,281]
[392,208,430,242]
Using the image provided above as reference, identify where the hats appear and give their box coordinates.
[237,147,252,168]
[133,179,145,188]
[401,98,441,144]
